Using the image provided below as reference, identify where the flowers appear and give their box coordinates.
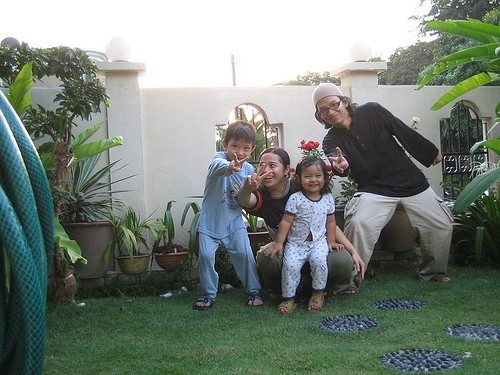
[402,117,420,159]
[289,140,336,190]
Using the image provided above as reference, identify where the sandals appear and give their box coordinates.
[278,298,297,315]
[308,291,327,312]
[247,293,264,307]
[340,286,356,294]
[433,274,451,283]
[197,295,217,311]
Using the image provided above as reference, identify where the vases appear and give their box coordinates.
[380,203,417,252]
[336,205,345,231]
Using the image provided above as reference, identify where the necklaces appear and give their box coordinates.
[271,179,290,199]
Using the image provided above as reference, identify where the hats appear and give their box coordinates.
[312,83,343,108]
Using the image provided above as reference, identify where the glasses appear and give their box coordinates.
[318,99,341,115]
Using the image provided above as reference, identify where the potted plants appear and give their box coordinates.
[53,152,140,278]
[241,210,271,256]
[151,200,189,270]
[102,205,167,275]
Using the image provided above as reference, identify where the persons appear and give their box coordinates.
[313,82,454,296]
[264,157,344,314]
[236,148,364,298]
[192,121,264,309]
